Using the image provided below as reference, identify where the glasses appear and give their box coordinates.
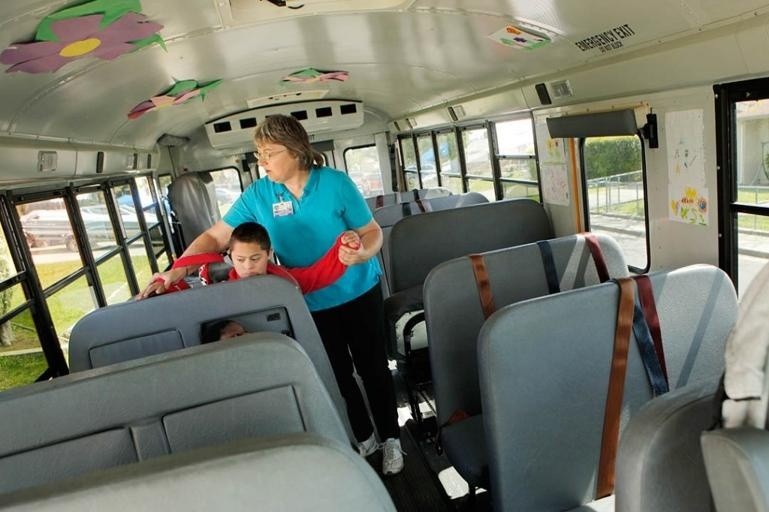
[253,149,283,161]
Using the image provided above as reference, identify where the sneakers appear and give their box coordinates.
[358,432,380,458]
[381,436,405,476]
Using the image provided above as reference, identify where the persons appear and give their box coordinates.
[132,223,360,302]
[201,321,250,343]
[148,114,408,474]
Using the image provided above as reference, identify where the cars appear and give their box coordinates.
[21,203,175,238]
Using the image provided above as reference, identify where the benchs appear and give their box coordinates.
[366,188,769,512]
[0,274,395,511]
